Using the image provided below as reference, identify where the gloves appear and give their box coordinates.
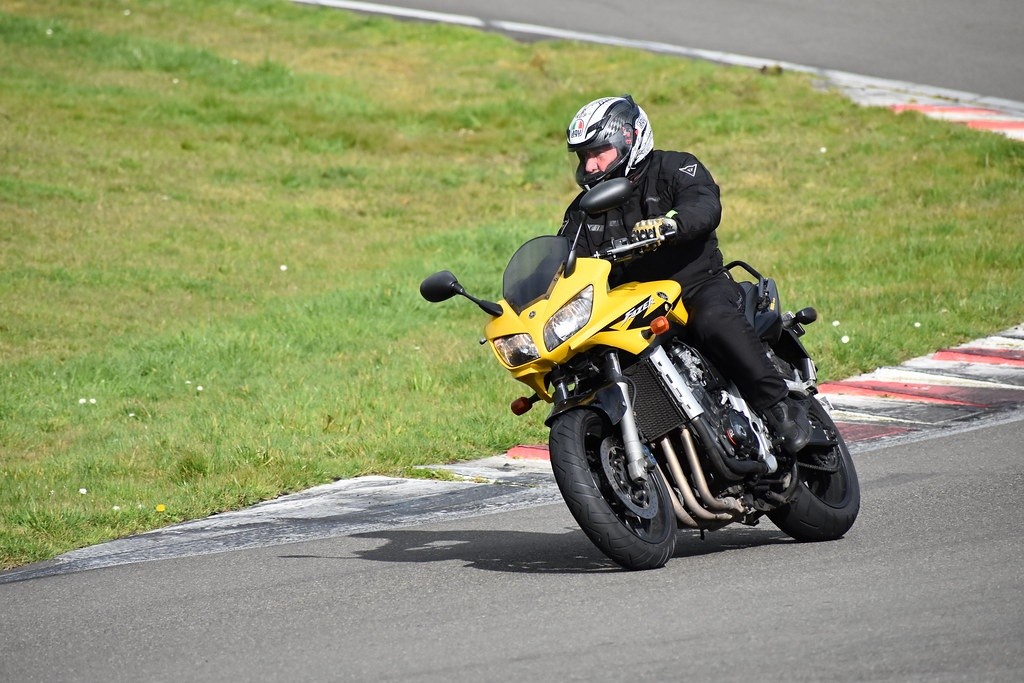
[631,218,679,256]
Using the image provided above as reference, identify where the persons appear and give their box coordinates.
[556,95,812,455]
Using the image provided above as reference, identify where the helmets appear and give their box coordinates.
[566,94,655,192]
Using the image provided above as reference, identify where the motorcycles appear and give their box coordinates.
[417,177,861,568]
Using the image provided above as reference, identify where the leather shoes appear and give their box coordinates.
[761,397,813,453]
